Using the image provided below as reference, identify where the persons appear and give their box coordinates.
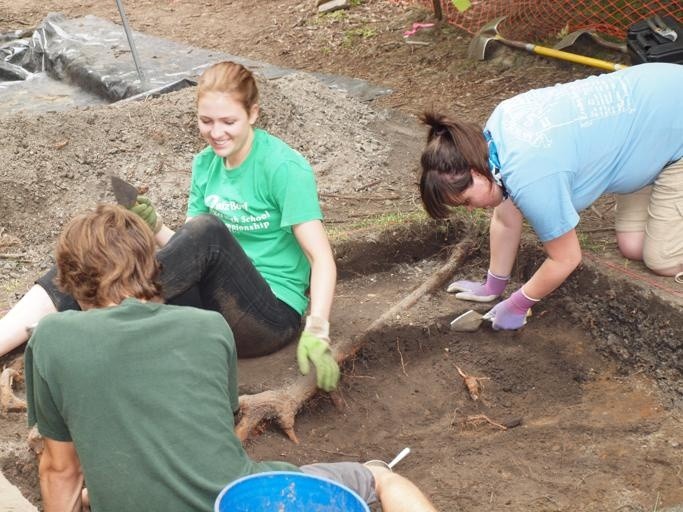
[24,204,437,512]
[0,61,339,393]
[419,62,683,331]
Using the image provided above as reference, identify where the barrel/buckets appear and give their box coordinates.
[211,473,375,512]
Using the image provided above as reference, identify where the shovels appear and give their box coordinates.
[449,305,532,333]
[552,24,628,54]
[467,13,622,72]
[364,446,414,472]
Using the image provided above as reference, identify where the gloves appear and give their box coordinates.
[132,196,161,233]
[447,271,508,303]
[482,289,539,332]
[297,317,340,391]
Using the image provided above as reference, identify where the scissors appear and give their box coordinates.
[645,13,679,43]
